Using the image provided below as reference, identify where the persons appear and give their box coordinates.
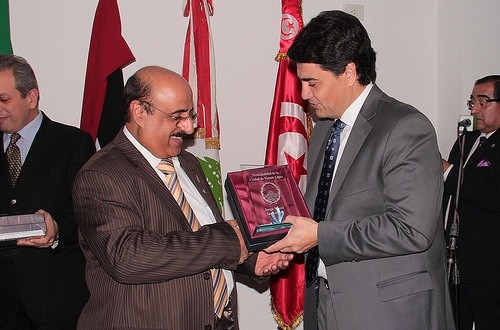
[442,75,500,330]
[0,55,97,330]
[262,10,455,330]
[74,65,293,330]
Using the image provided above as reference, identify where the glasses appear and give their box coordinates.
[142,100,198,124]
[469,99,498,108]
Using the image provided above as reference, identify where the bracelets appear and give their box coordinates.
[54,232,59,241]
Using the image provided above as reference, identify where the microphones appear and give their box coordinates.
[458,115,478,131]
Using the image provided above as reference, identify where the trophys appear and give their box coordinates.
[241,163,298,235]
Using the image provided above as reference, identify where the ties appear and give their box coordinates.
[304,118,347,289]
[5,132,22,189]
[479,136,487,150]
[157,157,229,320]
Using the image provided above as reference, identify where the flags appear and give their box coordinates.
[80,0,136,151]
[266,0,311,330]
[183,0,225,221]
